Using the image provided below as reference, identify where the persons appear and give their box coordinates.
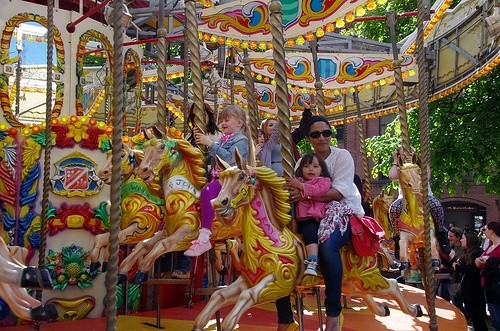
[435,222,500,331]
[172,102,225,280]
[254,95,313,176]
[389,146,448,260]
[184,105,255,257]
[285,152,331,276]
[276,116,365,331]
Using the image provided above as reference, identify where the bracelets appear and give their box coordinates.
[391,164,398,166]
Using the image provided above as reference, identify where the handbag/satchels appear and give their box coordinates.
[349,214,386,257]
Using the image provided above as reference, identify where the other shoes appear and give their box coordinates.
[285,320,300,331]
[172,271,190,279]
[184,239,212,256]
[304,259,318,277]
[340,313,344,331]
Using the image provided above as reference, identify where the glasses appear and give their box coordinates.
[308,130,332,138]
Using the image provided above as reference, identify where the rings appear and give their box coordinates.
[291,196,295,199]
